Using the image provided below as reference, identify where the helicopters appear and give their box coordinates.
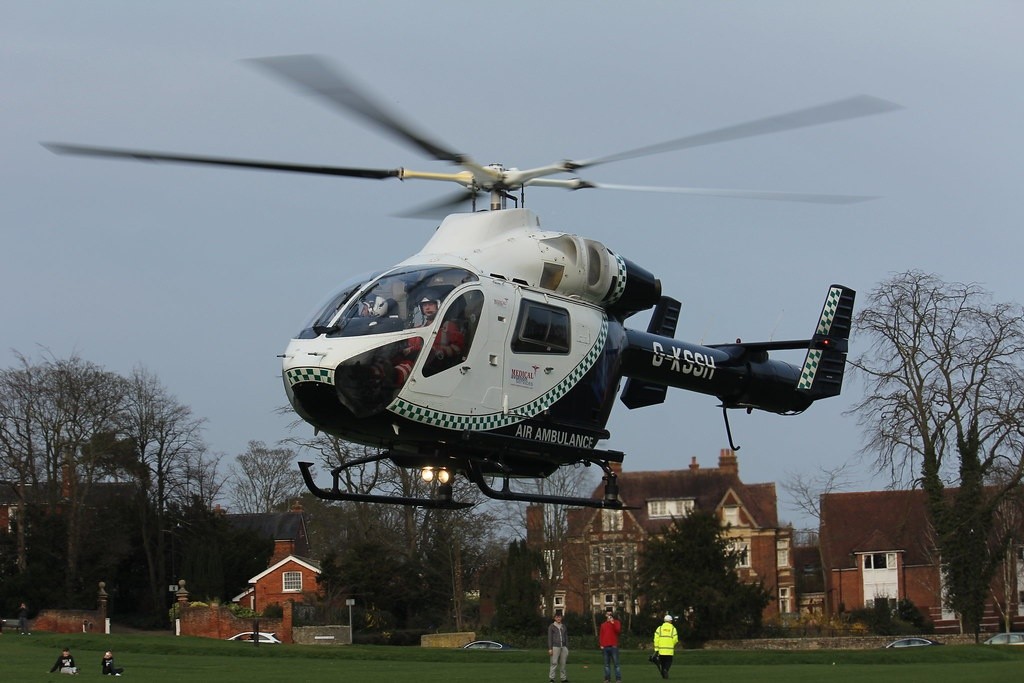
[40,53,904,510]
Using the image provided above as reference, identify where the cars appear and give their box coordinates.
[984,632,1024,644]
[458,640,517,649]
[883,637,945,649]
[227,631,281,643]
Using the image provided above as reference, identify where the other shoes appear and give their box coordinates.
[660,670,669,679]
[615,679,622,682]
[108,673,111,676]
[549,679,554,682]
[73,672,79,678]
[560,679,569,683]
[115,673,122,676]
[603,680,610,682]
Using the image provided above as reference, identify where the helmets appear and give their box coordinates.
[417,290,442,313]
[362,295,388,317]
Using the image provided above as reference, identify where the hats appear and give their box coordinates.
[664,614,672,621]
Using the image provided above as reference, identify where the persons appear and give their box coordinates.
[359,292,389,319]
[394,289,464,390]
[100,650,125,676]
[547,612,572,683]
[18,601,31,635]
[46,647,80,677]
[598,610,624,683]
[653,615,679,679]
